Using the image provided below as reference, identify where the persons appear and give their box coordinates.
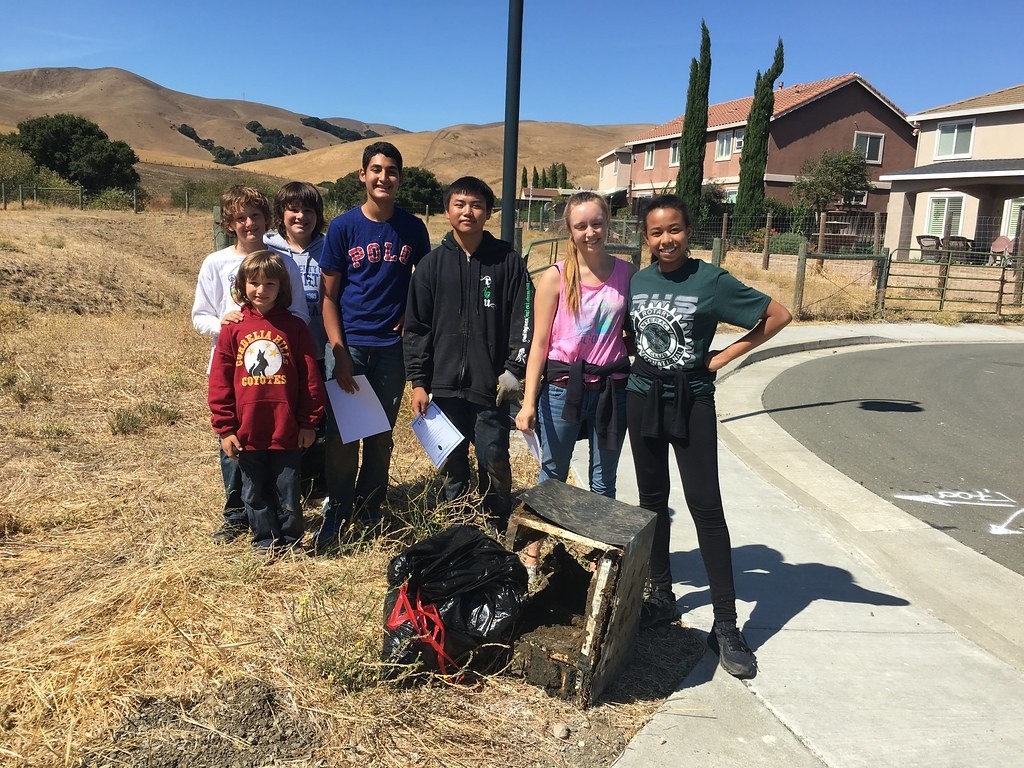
[311,142,431,548]
[627,195,792,679]
[191,184,310,543]
[208,249,325,547]
[404,175,537,544]
[263,181,330,508]
[515,192,640,584]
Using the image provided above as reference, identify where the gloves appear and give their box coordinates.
[496,370,522,406]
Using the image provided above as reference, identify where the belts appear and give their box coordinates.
[551,378,629,390]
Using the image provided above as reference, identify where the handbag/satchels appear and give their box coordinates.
[380,525,523,684]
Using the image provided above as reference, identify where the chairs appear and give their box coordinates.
[985,236,1012,267]
[941,236,975,264]
[916,235,943,263]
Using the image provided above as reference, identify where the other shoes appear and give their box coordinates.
[213,520,248,545]
[485,516,508,536]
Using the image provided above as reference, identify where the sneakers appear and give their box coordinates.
[706,618,761,678]
[641,591,678,627]
